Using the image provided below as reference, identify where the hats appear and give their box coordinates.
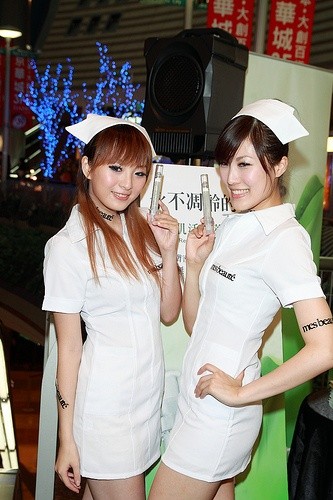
[64,113,156,159]
[231,98,310,145]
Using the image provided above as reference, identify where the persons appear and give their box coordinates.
[0,150,79,185]
[39,113,183,500]
[147,98,333,500]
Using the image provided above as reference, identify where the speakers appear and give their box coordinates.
[140,26,250,162]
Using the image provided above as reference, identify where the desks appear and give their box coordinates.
[288,387,333,500]
